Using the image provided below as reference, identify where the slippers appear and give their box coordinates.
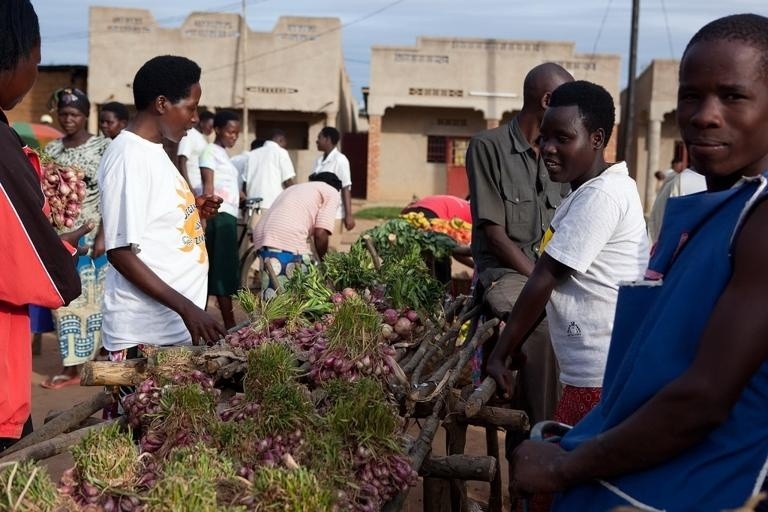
[41,374,81,388]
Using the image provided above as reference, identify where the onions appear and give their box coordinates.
[313,287,440,366]
[40,158,88,229]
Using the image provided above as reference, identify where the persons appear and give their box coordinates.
[99,56,230,440]
[648,164,708,243]
[399,193,472,284]
[229,138,264,200]
[239,129,296,232]
[315,127,356,231]
[177,112,215,198]
[484,80,655,512]
[0,0,82,452]
[654,170,666,193]
[665,158,682,181]
[466,63,572,424]
[507,12,767,512]
[44,86,105,385]
[197,111,246,332]
[99,102,129,139]
[253,172,342,302]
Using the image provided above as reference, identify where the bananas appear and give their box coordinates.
[397,211,473,234]
